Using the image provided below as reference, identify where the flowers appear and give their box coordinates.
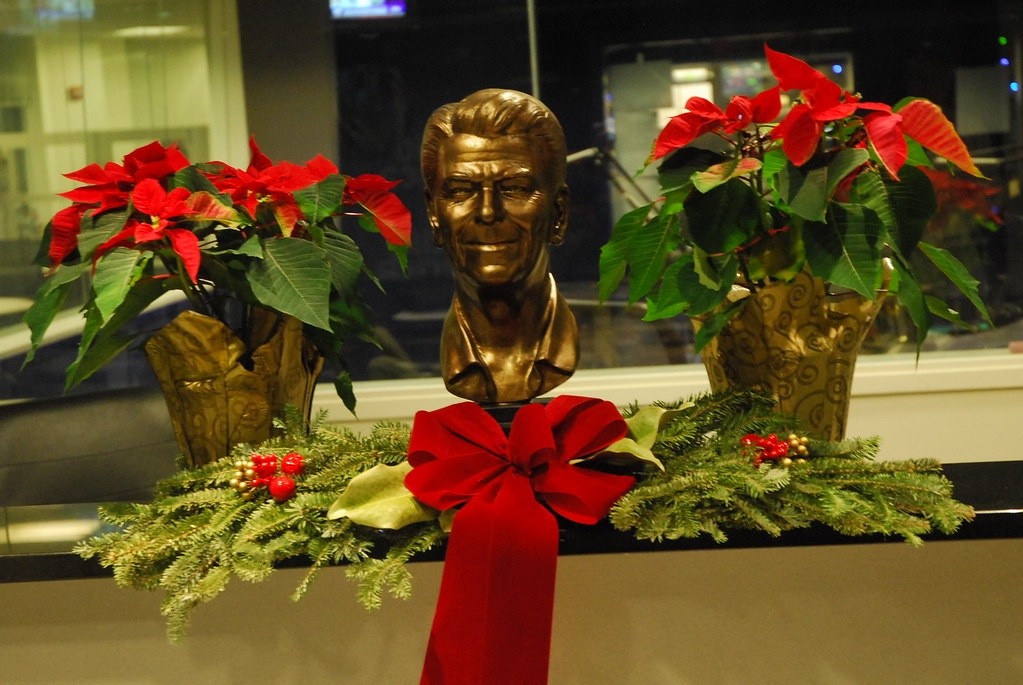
[20,135,413,422]
[600,41,993,371]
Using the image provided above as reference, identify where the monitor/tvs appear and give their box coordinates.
[590,27,869,250]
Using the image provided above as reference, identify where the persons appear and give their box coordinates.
[419,88,581,404]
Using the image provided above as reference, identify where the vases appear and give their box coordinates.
[146,305,325,471]
[674,258,895,448]
